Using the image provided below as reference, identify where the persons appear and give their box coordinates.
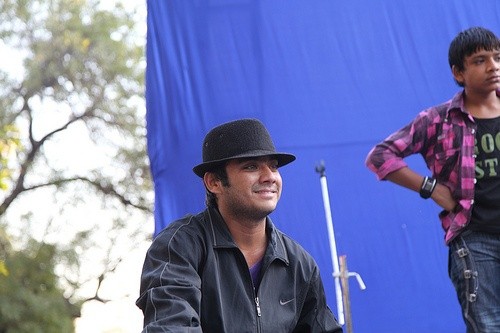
[135,119,346,333]
[362,27,500,333]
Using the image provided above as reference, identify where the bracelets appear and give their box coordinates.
[418,176,438,200]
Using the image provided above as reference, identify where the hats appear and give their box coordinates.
[192,118,297,178]
